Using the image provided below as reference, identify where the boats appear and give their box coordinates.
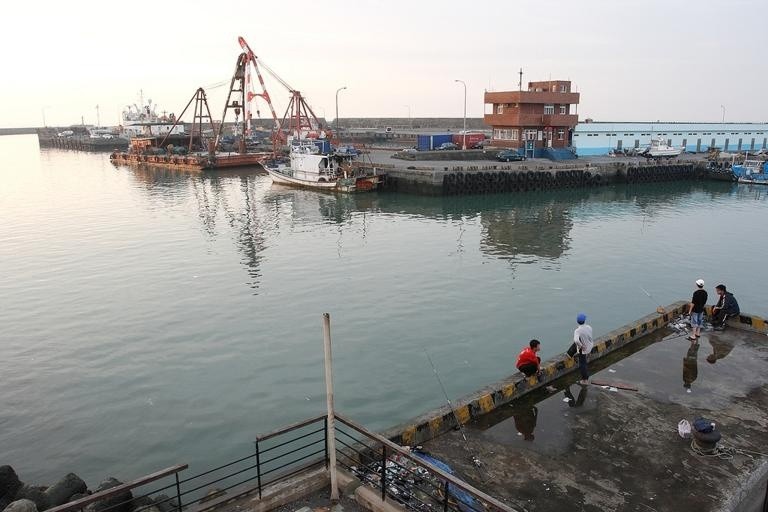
[256,126,387,195]
[624,147,647,156]
[731,159,768,185]
[642,138,686,160]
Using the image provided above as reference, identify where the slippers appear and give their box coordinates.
[687,337,695,340]
[695,334,700,337]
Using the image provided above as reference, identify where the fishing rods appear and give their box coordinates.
[639,287,688,334]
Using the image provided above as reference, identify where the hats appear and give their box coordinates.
[696,280,704,286]
[577,314,586,322]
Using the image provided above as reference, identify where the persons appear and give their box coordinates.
[565,314,594,385]
[510,404,539,443]
[688,279,708,340]
[705,332,735,365]
[711,285,740,331]
[680,334,700,391]
[515,340,546,382]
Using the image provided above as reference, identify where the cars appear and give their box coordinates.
[402,148,419,152]
[472,141,490,149]
[435,142,458,151]
[497,149,528,162]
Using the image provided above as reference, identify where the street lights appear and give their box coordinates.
[402,103,410,121]
[454,78,468,149]
[335,86,347,147]
[719,104,725,126]
